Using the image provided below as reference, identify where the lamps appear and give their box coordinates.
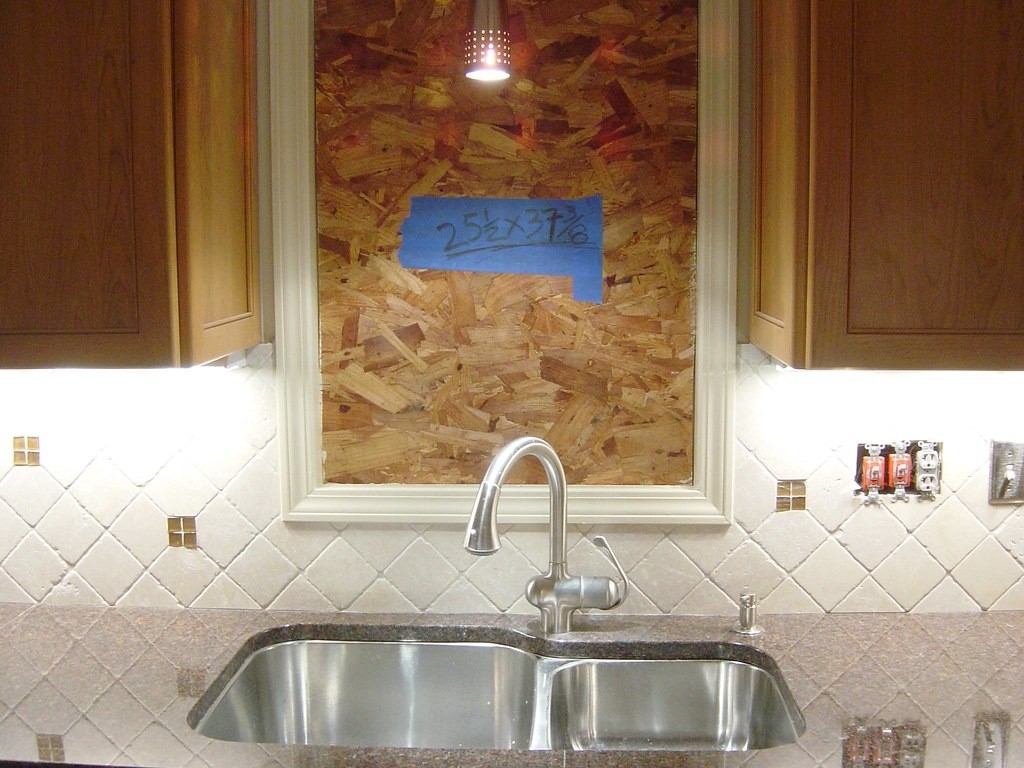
[463,0,512,81]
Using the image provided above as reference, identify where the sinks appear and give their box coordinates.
[549,638,808,754]
[183,622,544,752]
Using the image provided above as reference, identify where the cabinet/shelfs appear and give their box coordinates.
[749,0,1024,373]
[0,0,262,372]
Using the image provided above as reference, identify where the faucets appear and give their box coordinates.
[460,434,631,636]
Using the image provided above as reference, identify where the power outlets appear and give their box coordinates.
[916,450,940,493]
[896,725,927,768]
